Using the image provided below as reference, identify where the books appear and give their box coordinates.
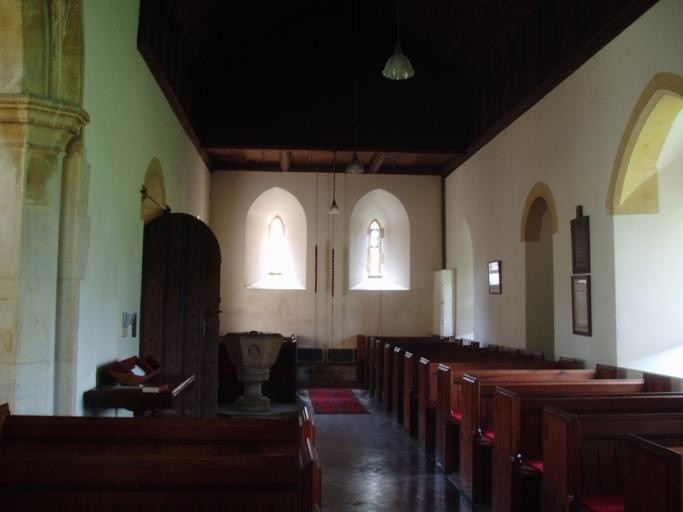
[141,383,169,393]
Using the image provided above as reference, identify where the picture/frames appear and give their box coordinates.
[486,260,503,294]
[570,275,591,336]
[570,215,592,273]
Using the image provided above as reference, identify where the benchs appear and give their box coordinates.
[1,403,320,512]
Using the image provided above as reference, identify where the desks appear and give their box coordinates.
[81,373,199,416]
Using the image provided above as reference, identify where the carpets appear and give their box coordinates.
[308,387,367,415]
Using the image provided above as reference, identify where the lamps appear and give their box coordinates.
[342,151,366,173]
[381,1,415,80]
[327,149,341,215]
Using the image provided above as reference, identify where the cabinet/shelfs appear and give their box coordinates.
[219,330,299,402]
